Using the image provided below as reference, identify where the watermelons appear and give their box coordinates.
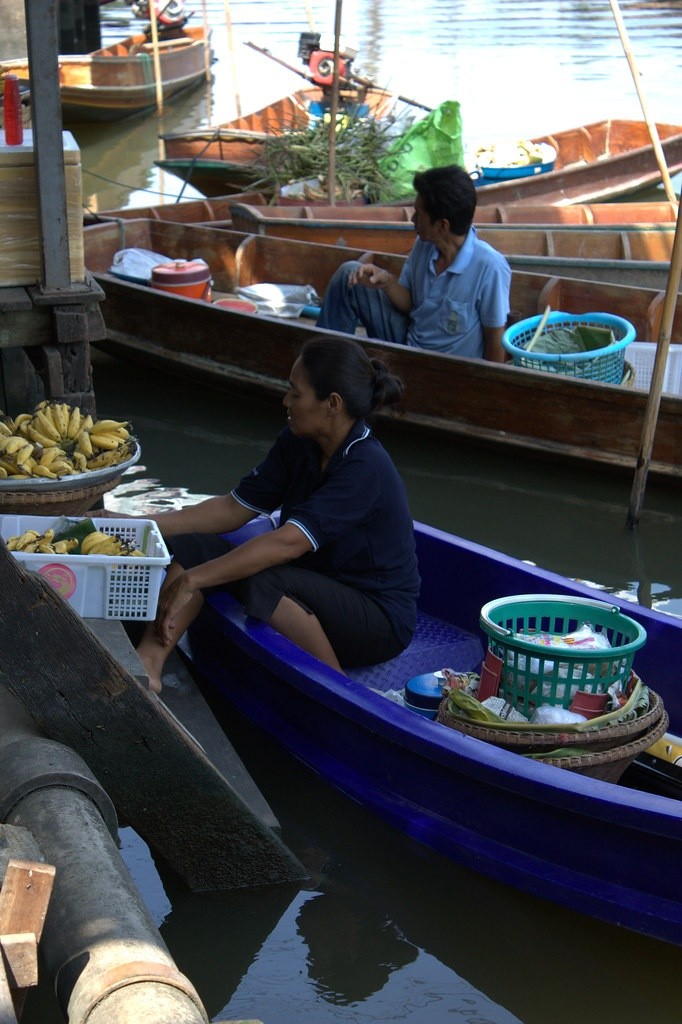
[211,298,258,314]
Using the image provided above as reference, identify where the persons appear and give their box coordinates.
[317,163,511,363]
[83,337,422,694]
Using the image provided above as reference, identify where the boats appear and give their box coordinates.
[82,219,682,479]
[80,119,682,232]
[226,202,682,294]
[153,82,400,198]
[0,25,217,121]
[161,505,682,940]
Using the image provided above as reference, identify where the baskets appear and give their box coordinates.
[532,708,671,786]
[0,514,173,621]
[439,686,665,760]
[481,592,649,722]
[500,309,638,386]
[0,474,121,518]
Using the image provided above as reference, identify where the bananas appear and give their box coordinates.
[0,399,145,557]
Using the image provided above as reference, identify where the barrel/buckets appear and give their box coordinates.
[403,671,449,722]
[151,258,213,304]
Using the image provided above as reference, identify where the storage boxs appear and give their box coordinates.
[502,310,636,386]
[0,400,141,516]
[0,513,174,623]
[476,594,648,725]
